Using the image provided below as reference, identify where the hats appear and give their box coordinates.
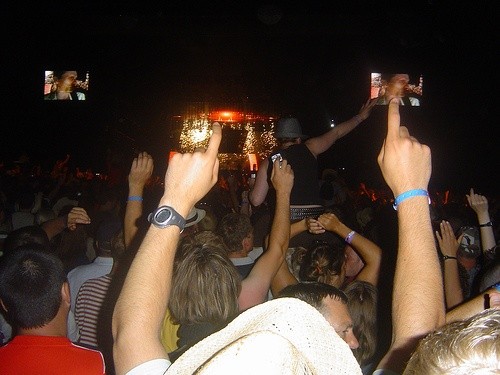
[356,207,375,225]
[95,216,122,240]
[184,206,206,227]
[455,225,480,255]
[53,198,80,217]
[272,117,309,138]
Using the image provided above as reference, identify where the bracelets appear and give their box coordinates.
[393,189,432,211]
[480,221,493,227]
[127,196,143,201]
[443,255,457,260]
[63,219,67,232]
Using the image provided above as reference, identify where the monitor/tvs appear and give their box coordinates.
[43,68,91,102]
[368,72,427,108]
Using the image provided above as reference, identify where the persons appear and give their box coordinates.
[44,70,85,100]
[0,95,500,374]
[371,73,420,106]
[378,86,385,97]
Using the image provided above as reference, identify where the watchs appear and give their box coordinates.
[345,231,355,243]
[147,206,186,232]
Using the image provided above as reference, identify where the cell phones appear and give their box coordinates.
[272,153,283,168]
